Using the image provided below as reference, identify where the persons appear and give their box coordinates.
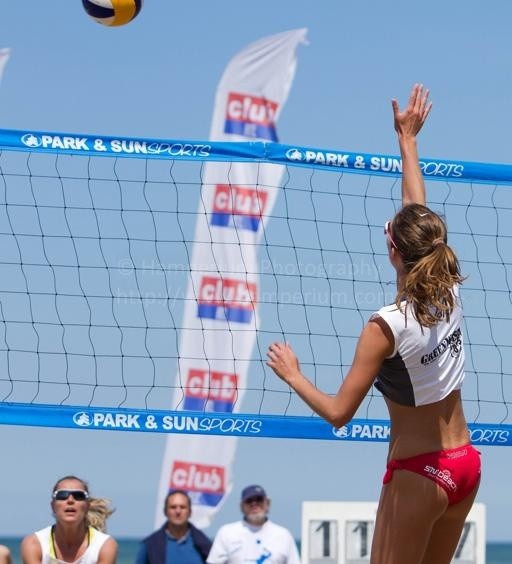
[19,477,119,564]
[0,541,16,562]
[134,489,212,564]
[266,81,484,564]
[203,485,299,563]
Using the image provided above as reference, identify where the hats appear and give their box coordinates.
[242,486,265,501]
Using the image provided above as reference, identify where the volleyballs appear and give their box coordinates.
[83,0,142,26]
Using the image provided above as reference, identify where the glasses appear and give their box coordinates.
[53,488,90,500]
[384,221,404,256]
